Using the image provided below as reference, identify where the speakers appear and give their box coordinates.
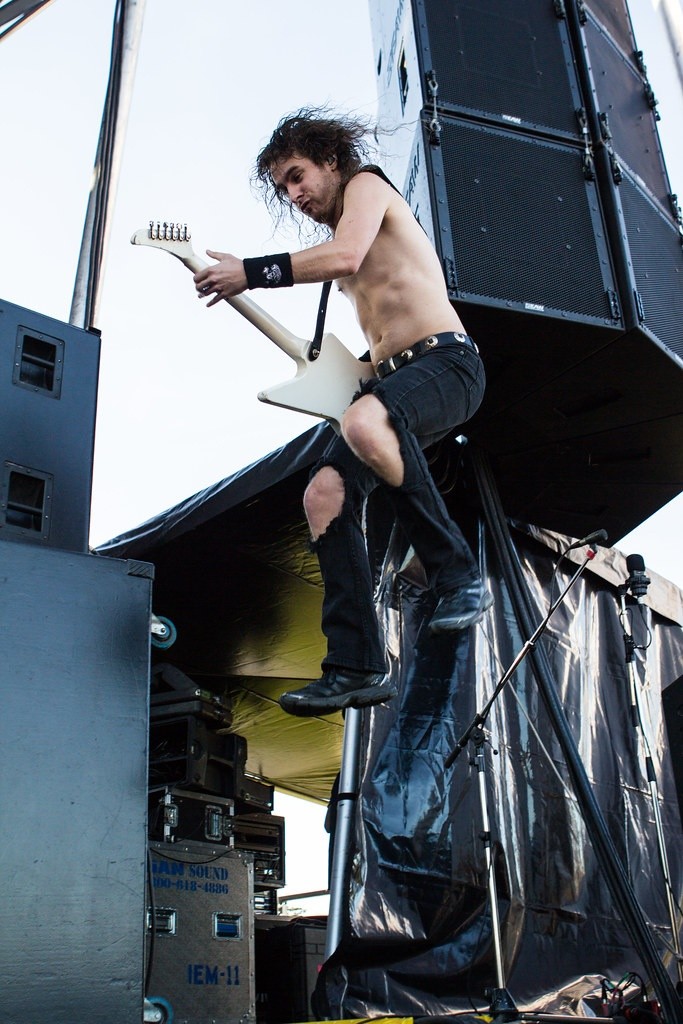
[0,296,101,553]
[371,0,683,554]
[254,918,308,1024]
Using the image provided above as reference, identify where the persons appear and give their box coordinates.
[193,99,494,717]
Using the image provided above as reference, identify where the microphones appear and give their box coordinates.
[625,555,648,612]
[570,528,609,550]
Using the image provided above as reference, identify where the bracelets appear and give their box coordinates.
[243,252,294,290]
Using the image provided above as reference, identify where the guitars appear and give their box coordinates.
[131,218,379,435]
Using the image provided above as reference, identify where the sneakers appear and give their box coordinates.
[429,574,497,631]
[276,664,397,719]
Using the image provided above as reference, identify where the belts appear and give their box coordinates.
[369,329,481,383]
